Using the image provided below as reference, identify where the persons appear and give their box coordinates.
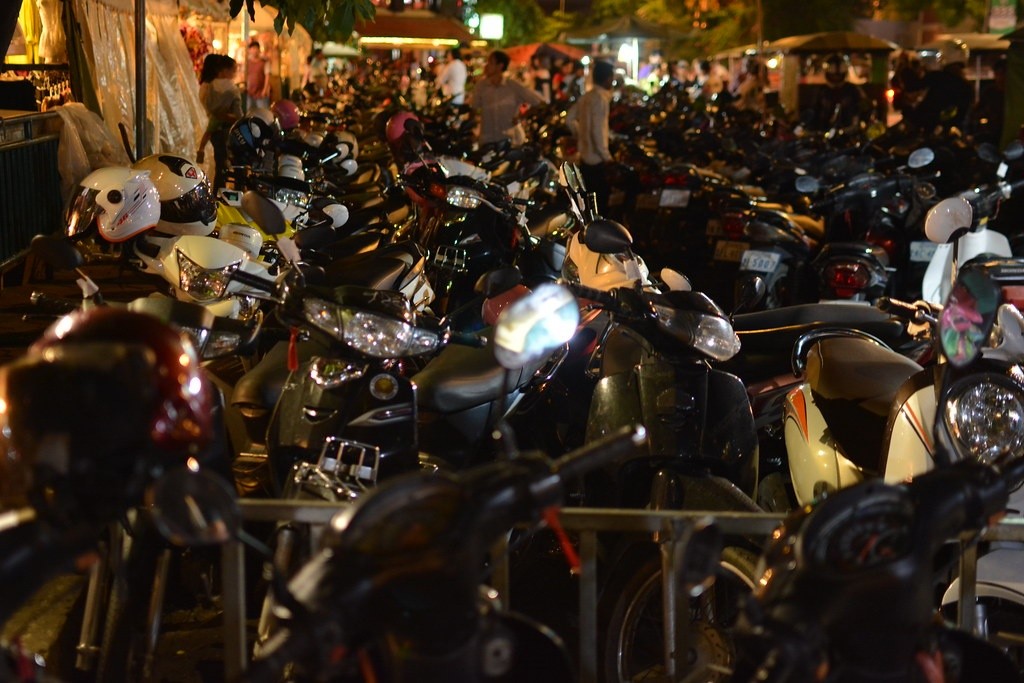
[434,49,468,105]
[246,41,272,109]
[890,40,1008,149]
[462,51,639,233]
[196,53,247,200]
[701,61,723,99]
[813,56,872,149]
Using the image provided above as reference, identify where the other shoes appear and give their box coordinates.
[196,151,204,164]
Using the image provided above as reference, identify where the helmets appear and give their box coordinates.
[385,111,424,150]
[132,154,218,238]
[227,108,278,153]
[271,99,300,130]
[318,130,359,162]
[561,229,650,290]
[61,166,161,240]
[4,305,210,461]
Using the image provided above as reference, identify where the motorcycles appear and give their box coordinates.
[575,75,789,170]
[347,110,660,322]
[49,113,434,354]
[630,90,1024,313]
[274,56,595,159]
[0,284,1024,683]
